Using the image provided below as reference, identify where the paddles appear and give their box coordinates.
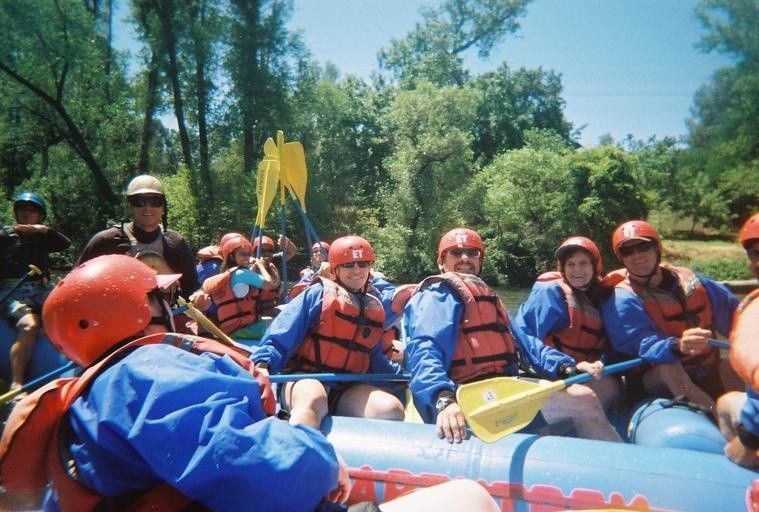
[256,160,279,259]
[457,359,643,443]
[282,141,312,261]
[175,297,258,357]
[250,155,278,246]
[276,130,288,302]
[263,138,326,274]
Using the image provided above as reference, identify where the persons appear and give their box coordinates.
[0,253,503,512]
[603,220,745,420]
[73,176,330,341]
[1,192,72,401]
[510,236,624,412]
[716,211,759,469]
[250,234,406,432]
[402,227,625,443]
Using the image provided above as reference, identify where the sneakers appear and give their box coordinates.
[3,389,28,408]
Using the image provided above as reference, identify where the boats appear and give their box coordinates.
[319,395,759,512]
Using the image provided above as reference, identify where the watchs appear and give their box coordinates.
[435,396,455,412]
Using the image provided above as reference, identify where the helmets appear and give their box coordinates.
[126,175,164,197]
[14,192,48,217]
[220,233,245,255]
[312,242,330,255]
[739,213,759,248]
[554,237,600,264]
[252,235,275,252]
[42,254,183,369]
[222,237,252,263]
[612,220,663,257]
[438,229,484,264]
[329,235,376,270]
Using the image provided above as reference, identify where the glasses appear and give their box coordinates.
[261,249,273,253]
[445,247,482,260]
[127,197,165,208]
[237,250,252,257]
[340,261,369,268]
[619,240,657,257]
[748,247,759,260]
